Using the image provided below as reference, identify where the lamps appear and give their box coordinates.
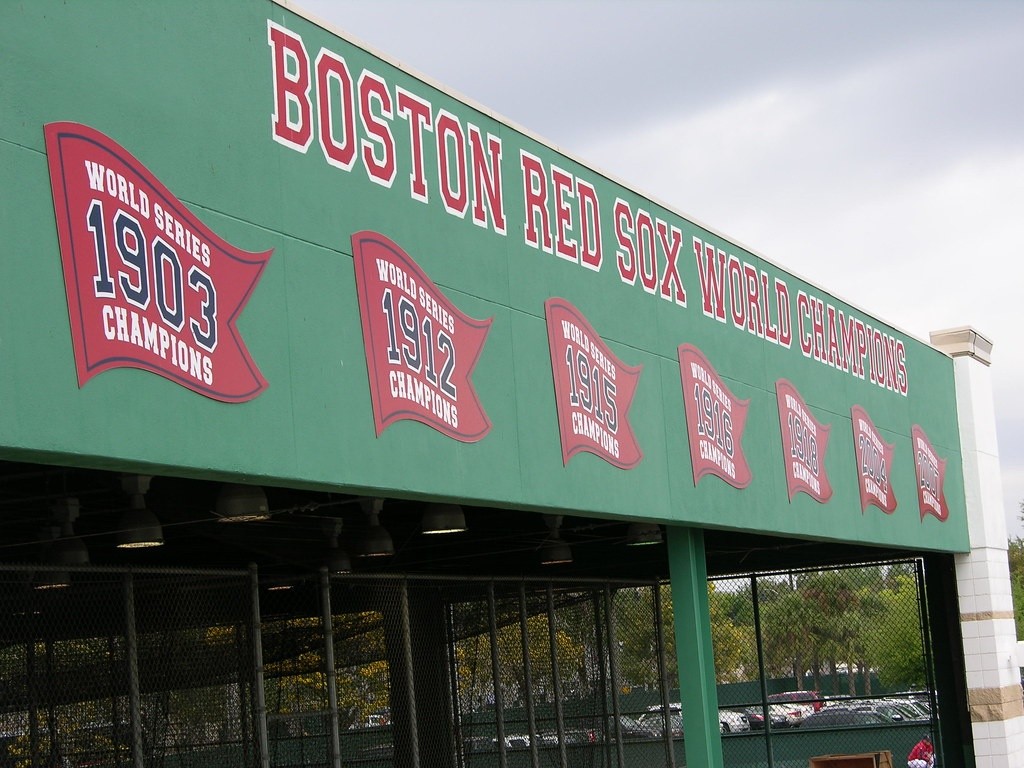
[28,483,573,594]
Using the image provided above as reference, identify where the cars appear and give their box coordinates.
[639,716,684,738]
[595,715,662,740]
[467,728,605,750]
[738,689,938,730]
[348,715,388,729]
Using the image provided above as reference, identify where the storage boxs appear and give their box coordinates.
[807,750,892,768]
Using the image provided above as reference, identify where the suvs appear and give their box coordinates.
[642,703,752,734]
